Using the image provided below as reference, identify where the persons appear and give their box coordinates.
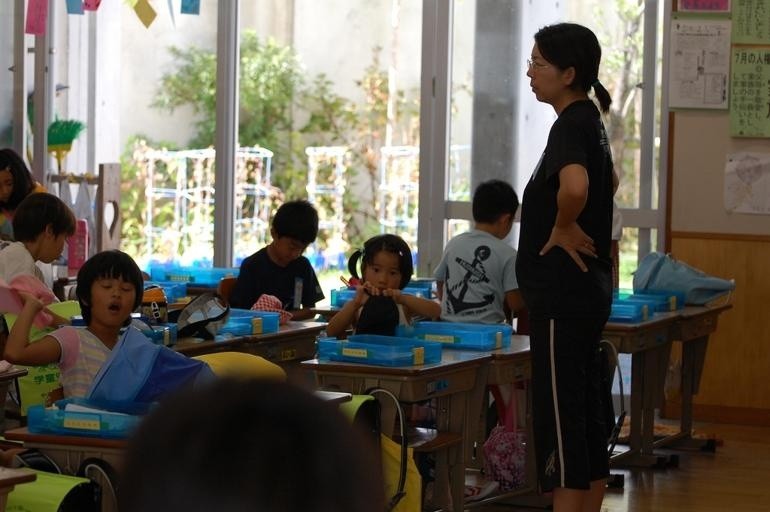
[4,250,143,399]
[1,192,76,313]
[432,179,527,326]
[516,23,620,512]
[229,201,325,321]
[326,234,442,337]
[0,148,50,241]
[110,373,389,512]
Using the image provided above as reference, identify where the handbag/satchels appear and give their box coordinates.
[483,427,526,491]
[634,252,735,305]
[86,326,217,416]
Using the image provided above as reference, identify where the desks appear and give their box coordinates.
[1,264,736,512]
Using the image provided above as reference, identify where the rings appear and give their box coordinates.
[585,242,589,247]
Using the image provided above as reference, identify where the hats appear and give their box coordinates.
[174,291,230,338]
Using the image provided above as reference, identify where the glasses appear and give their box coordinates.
[527,59,552,73]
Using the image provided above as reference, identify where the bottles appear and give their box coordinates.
[141,283,167,326]
[68,219,89,276]
